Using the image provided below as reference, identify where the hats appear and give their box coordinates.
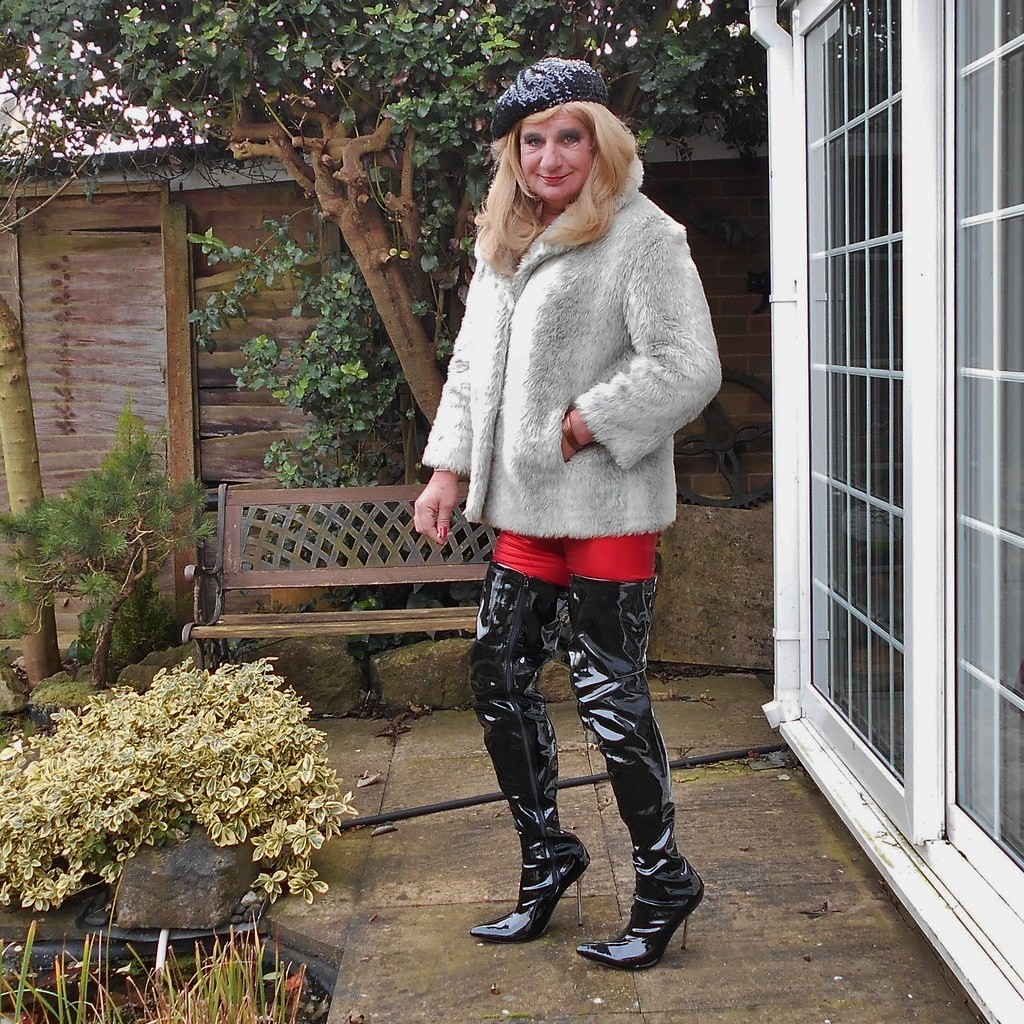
[490,57,609,140]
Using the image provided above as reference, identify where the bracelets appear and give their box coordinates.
[562,412,584,451]
[433,468,451,472]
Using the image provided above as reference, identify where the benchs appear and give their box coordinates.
[179,482,500,672]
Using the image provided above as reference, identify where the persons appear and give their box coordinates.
[415,56,722,972]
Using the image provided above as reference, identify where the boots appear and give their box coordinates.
[469,560,592,943]
[571,553,700,964]
